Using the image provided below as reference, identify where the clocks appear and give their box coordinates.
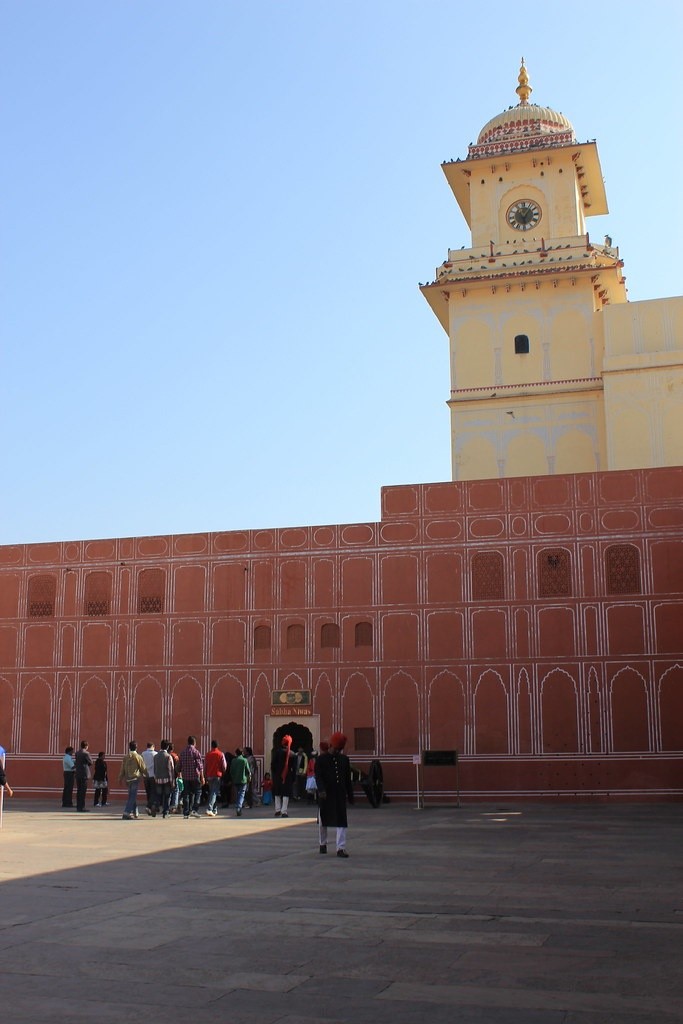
[505,199,543,232]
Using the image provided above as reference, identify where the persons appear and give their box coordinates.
[314,732,354,857]
[0,761,13,797]
[75,740,93,812]
[62,747,76,808]
[117,741,149,820]
[141,735,317,819]
[270,735,297,817]
[92,752,111,807]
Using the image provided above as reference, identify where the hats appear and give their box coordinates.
[330,732,347,749]
[282,735,292,784]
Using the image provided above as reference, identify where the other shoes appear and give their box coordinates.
[243,804,251,808]
[122,814,134,819]
[256,798,261,807]
[184,815,188,818]
[274,811,281,817]
[94,803,101,807]
[190,810,201,817]
[104,802,111,806]
[337,849,349,858]
[320,845,327,854]
[282,813,288,817]
[237,808,241,816]
[77,807,90,811]
[206,808,217,816]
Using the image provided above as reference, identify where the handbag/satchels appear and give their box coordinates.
[93,780,107,788]
